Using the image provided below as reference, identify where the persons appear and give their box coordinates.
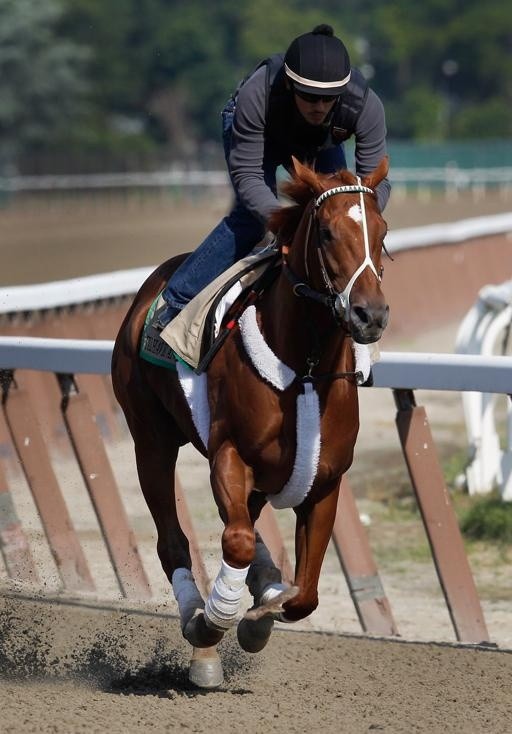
[134,20,394,388]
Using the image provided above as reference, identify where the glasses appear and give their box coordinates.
[294,90,336,103]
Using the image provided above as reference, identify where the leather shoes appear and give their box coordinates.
[152,304,180,333]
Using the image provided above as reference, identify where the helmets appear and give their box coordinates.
[282,24,352,96]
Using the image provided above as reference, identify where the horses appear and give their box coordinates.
[110,154,391,690]
